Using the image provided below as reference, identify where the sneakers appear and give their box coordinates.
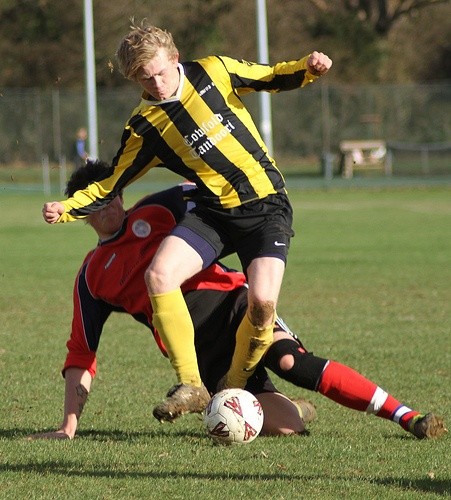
[216,375,228,393]
[415,413,448,439]
[152,382,211,424]
[291,398,316,423]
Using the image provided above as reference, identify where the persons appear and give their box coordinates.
[37,25,333,425]
[66,123,93,177]
[19,158,448,441]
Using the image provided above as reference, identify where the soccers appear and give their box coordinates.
[205,387,265,445]
